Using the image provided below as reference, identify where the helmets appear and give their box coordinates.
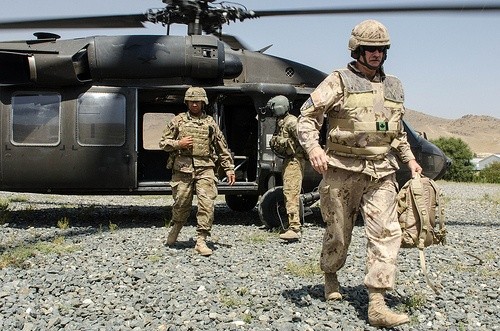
[184,86,209,106]
[267,96,291,118]
[349,21,391,70]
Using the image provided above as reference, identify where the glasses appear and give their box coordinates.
[363,45,385,52]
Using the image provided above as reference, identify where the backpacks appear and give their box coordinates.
[398,173,447,248]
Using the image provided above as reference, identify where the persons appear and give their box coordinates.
[267,94,309,243]
[159,86,236,256]
[296,19,422,327]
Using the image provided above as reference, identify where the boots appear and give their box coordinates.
[324,270,342,300]
[368,289,410,328]
[196,234,213,256]
[167,224,183,246]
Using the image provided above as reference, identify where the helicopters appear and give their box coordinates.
[0,0,500,229]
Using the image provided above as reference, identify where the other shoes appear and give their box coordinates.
[280,228,302,240]
